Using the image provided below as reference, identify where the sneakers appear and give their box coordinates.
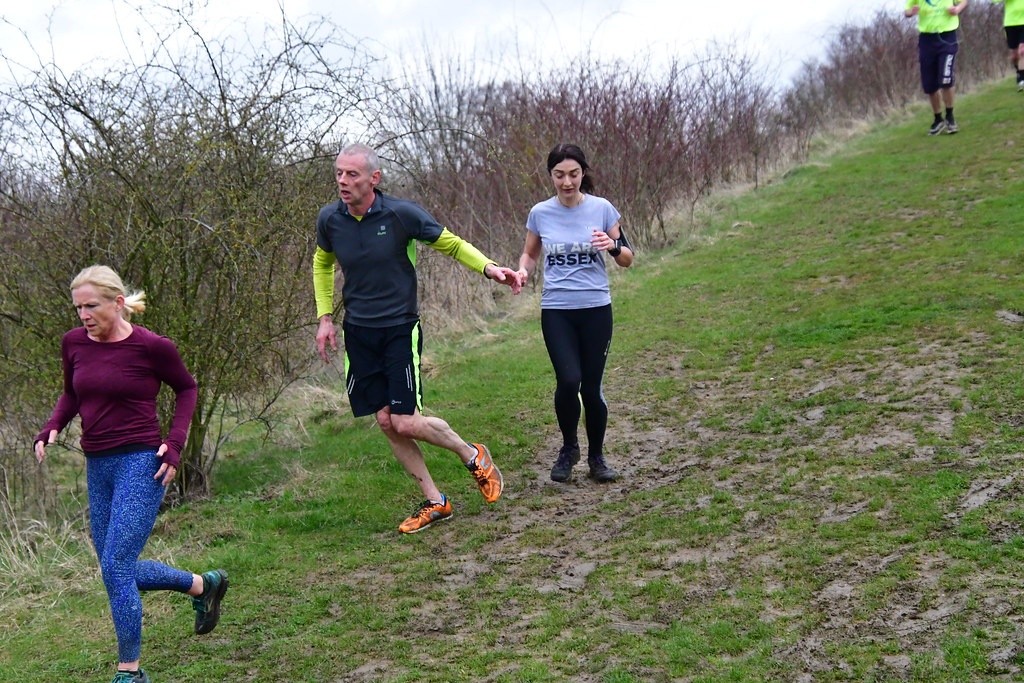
[463,443,503,504]
[112,668,148,683]
[192,569,230,635]
[945,118,960,134]
[1017,79,1024,92]
[398,493,453,534]
[587,455,616,482]
[550,445,581,482]
[928,119,946,135]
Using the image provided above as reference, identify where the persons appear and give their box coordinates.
[904,0,969,136]
[33,263,229,683]
[313,141,521,534]
[514,144,635,482]
[993,0,1024,93]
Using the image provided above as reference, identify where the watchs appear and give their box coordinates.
[607,239,621,257]
